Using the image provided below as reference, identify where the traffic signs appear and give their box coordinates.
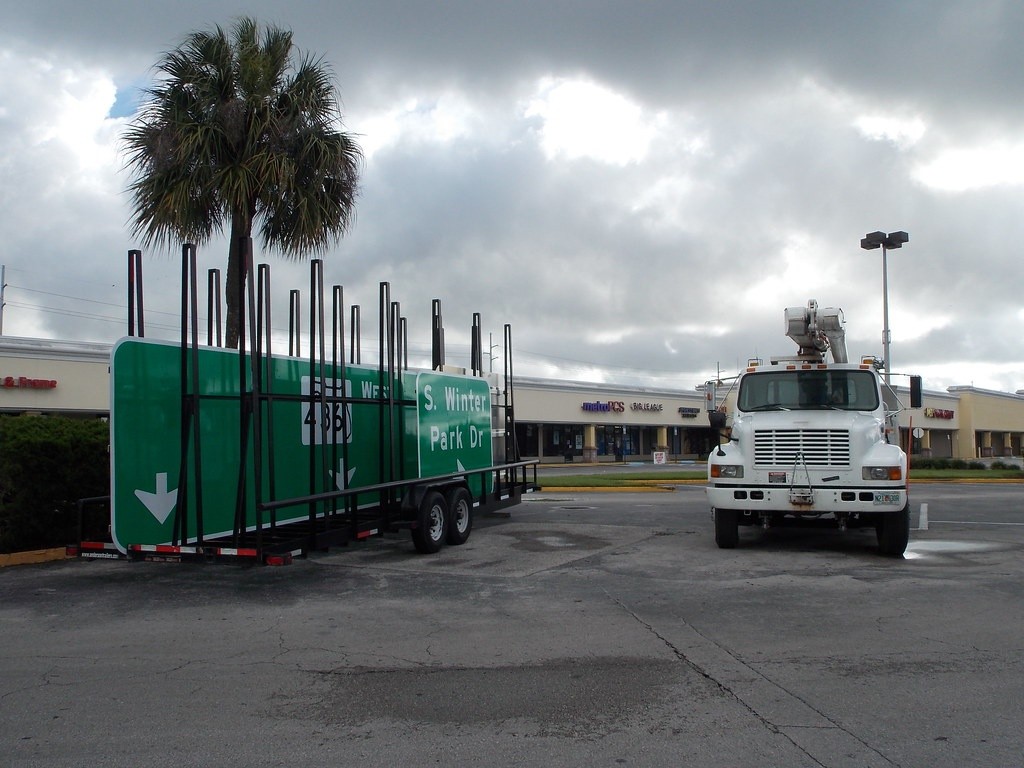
[110,336,493,556]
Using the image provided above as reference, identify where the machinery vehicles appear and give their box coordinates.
[703,298,923,554]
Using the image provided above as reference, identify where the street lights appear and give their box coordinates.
[860,230,909,384]
